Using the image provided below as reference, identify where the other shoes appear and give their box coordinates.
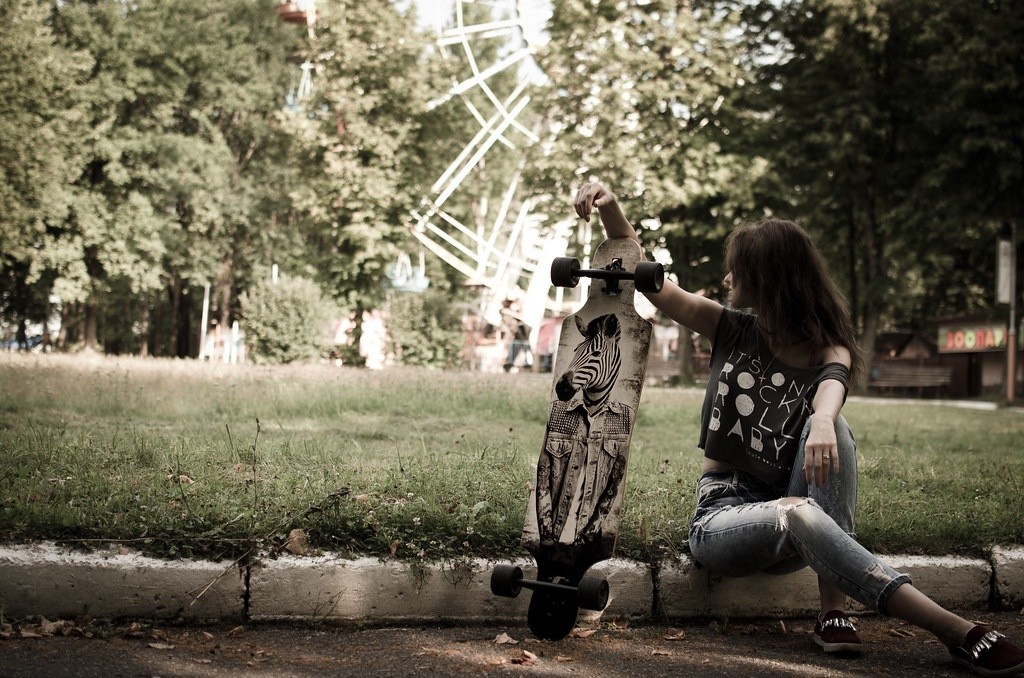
[813,610,861,653]
[950,620,1024,673]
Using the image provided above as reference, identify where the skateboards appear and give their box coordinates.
[484,229,675,647]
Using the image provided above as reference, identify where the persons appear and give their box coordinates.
[574,182,1023,670]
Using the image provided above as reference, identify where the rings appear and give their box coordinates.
[822,455,829,460]
